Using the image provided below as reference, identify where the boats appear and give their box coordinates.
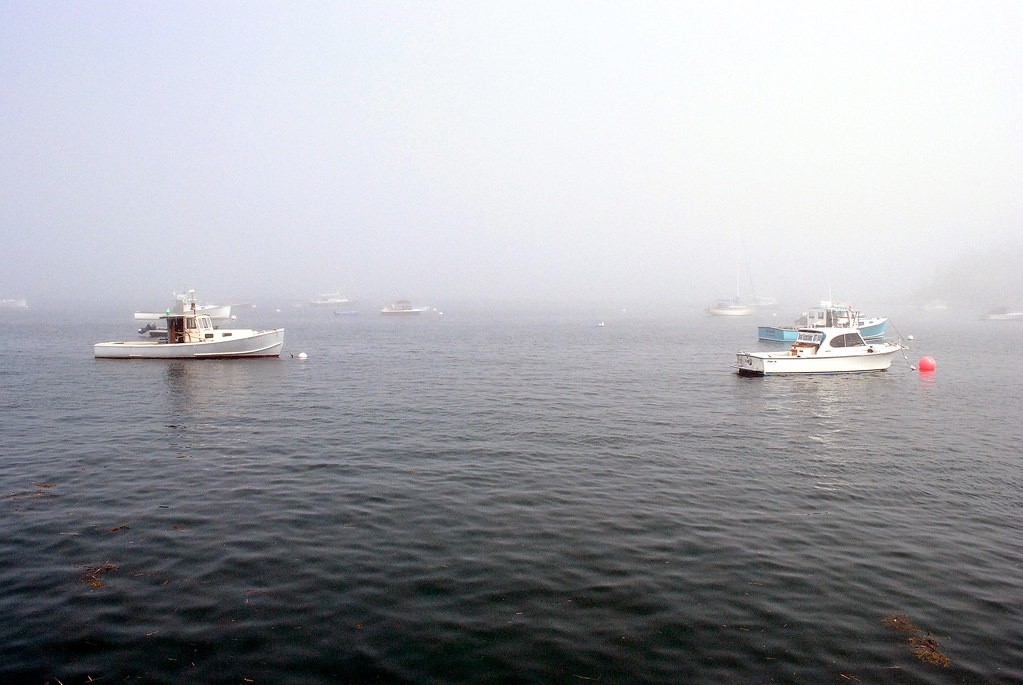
[0,300,28,308]
[988,312,1022,320]
[135,290,230,321]
[310,292,348,305]
[743,294,779,310]
[757,289,889,343]
[93,307,285,358]
[707,296,749,316]
[735,326,917,377]
[379,301,427,315]
[333,298,360,317]
